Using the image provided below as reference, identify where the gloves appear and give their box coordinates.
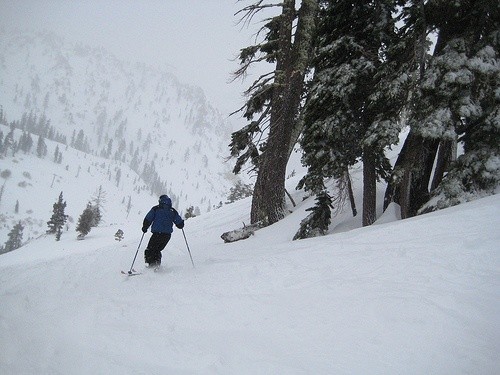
[141,227,147,233]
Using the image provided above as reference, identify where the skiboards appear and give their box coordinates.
[122,267,163,275]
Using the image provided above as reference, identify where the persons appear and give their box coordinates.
[141,194,184,269]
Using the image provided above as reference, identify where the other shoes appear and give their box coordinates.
[148,259,160,266]
